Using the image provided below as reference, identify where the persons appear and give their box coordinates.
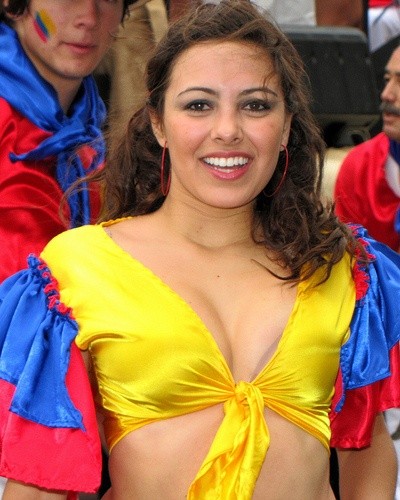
[0,0,134,282]
[332,45,400,256]
[0,0,400,500]
